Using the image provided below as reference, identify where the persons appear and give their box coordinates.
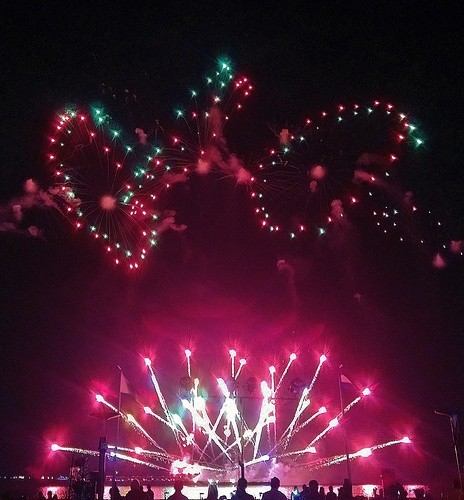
[205,484,220,500]
[442,479,464,500]
[326,485,338,500]
[168,480,188,500]
[109,481,121,500]
[146,484,154,500]
[126,478,150,500]
[52,494,59,500]
[291,480,327,500]
[47,491,53,500]
[231,477,254,500]
[261,477,287,500]
